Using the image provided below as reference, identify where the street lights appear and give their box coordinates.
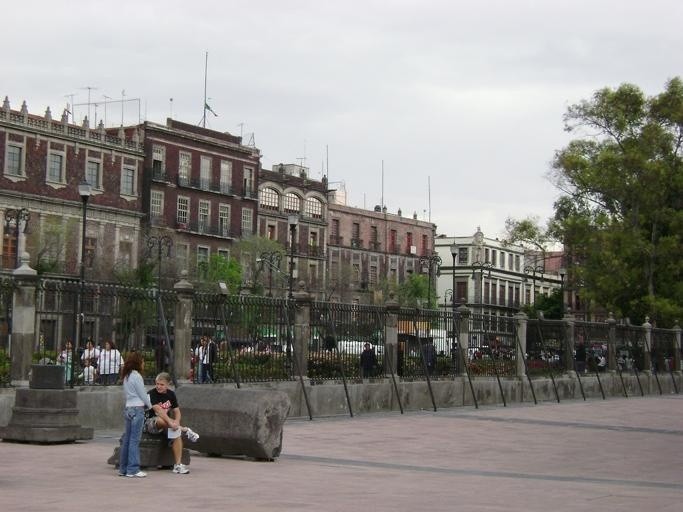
[446,239,460,367]
[470,260,492,350]
[70,175,92,349]
[3,205,31,277]
[521,264,546,320]
[419,253,442,357]
[144,233,176,372]
[281,214,299,379]
[258,249,283,298]
[558,267,567,322]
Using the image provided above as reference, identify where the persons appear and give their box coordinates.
[156,335,218,384]
[142,372,200,474]
[420,337,437,376]
[57,339,124,385]
[360,343,378,377]
[117,349,152,478]
[575,344,676,373]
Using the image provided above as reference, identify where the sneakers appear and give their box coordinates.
[125,470,147,479]
[183,426,200,443]
[119,469,125,478]
[171,463,189,474]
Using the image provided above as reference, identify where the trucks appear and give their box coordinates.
[338,341,384,357]
